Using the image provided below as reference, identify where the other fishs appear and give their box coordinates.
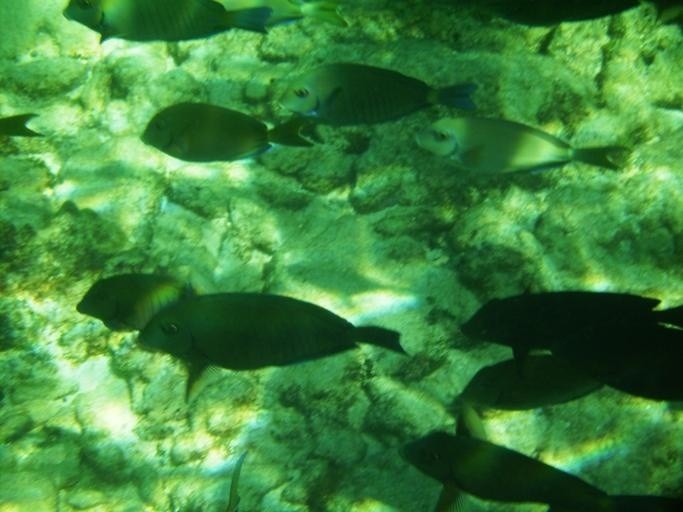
[135,289,411,407]
